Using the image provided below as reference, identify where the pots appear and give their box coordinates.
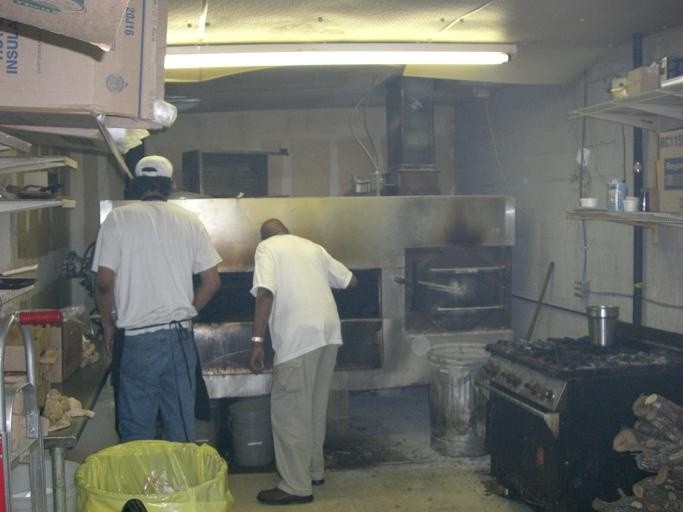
[585,304,619,348]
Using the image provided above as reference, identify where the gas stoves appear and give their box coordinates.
[484,335,683,379]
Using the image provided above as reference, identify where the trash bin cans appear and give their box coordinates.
[227,401,272,466]
[74,440,234,512]
[426,341,489,457]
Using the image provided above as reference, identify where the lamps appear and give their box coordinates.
[164,43,518,70]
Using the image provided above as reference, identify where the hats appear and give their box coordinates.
[135,155,173,178]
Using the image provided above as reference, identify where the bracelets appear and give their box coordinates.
[250,337,266,344]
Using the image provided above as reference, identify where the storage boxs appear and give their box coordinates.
[0,0,168,154]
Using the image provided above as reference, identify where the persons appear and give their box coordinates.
[246,218,359,506]
[91,154,223,444]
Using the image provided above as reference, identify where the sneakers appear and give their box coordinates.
[312,479,324,486]
[257,487,313,505]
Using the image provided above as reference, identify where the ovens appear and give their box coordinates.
[474,352,570,512]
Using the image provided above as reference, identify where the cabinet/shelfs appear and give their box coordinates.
[0,156,77,214]
[566,76,683,244]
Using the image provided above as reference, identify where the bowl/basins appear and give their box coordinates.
[579,197,600,208]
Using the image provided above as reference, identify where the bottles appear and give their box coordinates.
[639,191,649,212]
